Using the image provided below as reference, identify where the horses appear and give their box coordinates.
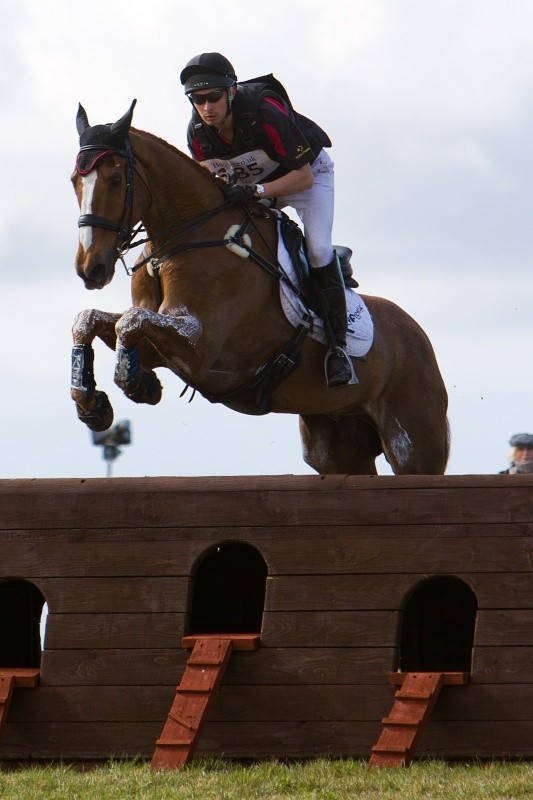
[70,98,451,475]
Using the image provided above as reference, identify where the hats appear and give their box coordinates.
[509,433,533,446]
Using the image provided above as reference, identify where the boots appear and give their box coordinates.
[309,249,352,387]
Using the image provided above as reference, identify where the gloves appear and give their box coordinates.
[224,183,263,208]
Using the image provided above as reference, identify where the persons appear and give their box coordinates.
[499,434,533,474]
[180,52,351,387]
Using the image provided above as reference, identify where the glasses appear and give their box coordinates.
[186,87,228,105]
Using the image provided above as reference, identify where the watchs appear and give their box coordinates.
[255,183,264,199]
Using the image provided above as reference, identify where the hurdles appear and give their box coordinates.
[2,478,531,765]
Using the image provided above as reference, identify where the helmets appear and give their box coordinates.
[180,53,237,95]
